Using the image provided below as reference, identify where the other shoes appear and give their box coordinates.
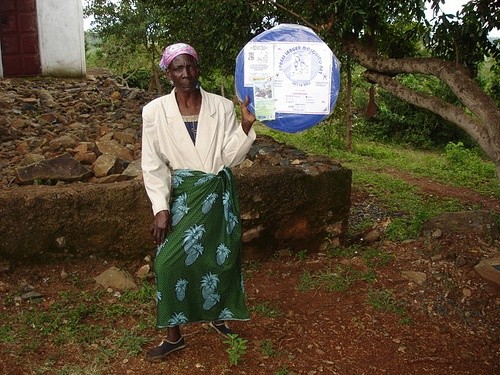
[209,320,235,340]
[148,335,186,359]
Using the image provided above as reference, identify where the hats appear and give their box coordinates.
[159,43,199,71]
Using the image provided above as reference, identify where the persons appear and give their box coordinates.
[142,43,257,359]
